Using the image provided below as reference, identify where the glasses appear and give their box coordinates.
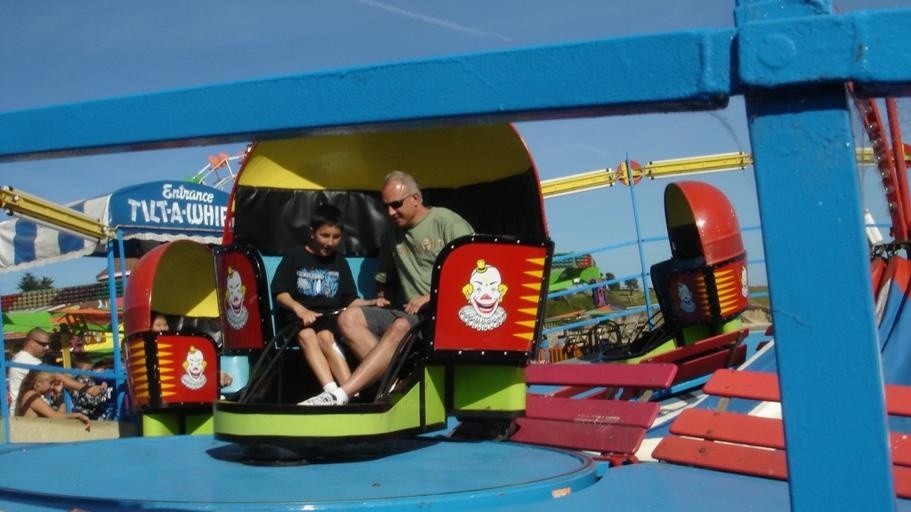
[33,339,49,346]
[382,194,412,209]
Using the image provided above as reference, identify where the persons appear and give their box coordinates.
[267,203,394,406]
[4,326,116,432]
[151,312,170,332]
[292,170,481,408]
[538,334,550,362]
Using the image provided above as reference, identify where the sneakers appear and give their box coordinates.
[296,392,338,406]
